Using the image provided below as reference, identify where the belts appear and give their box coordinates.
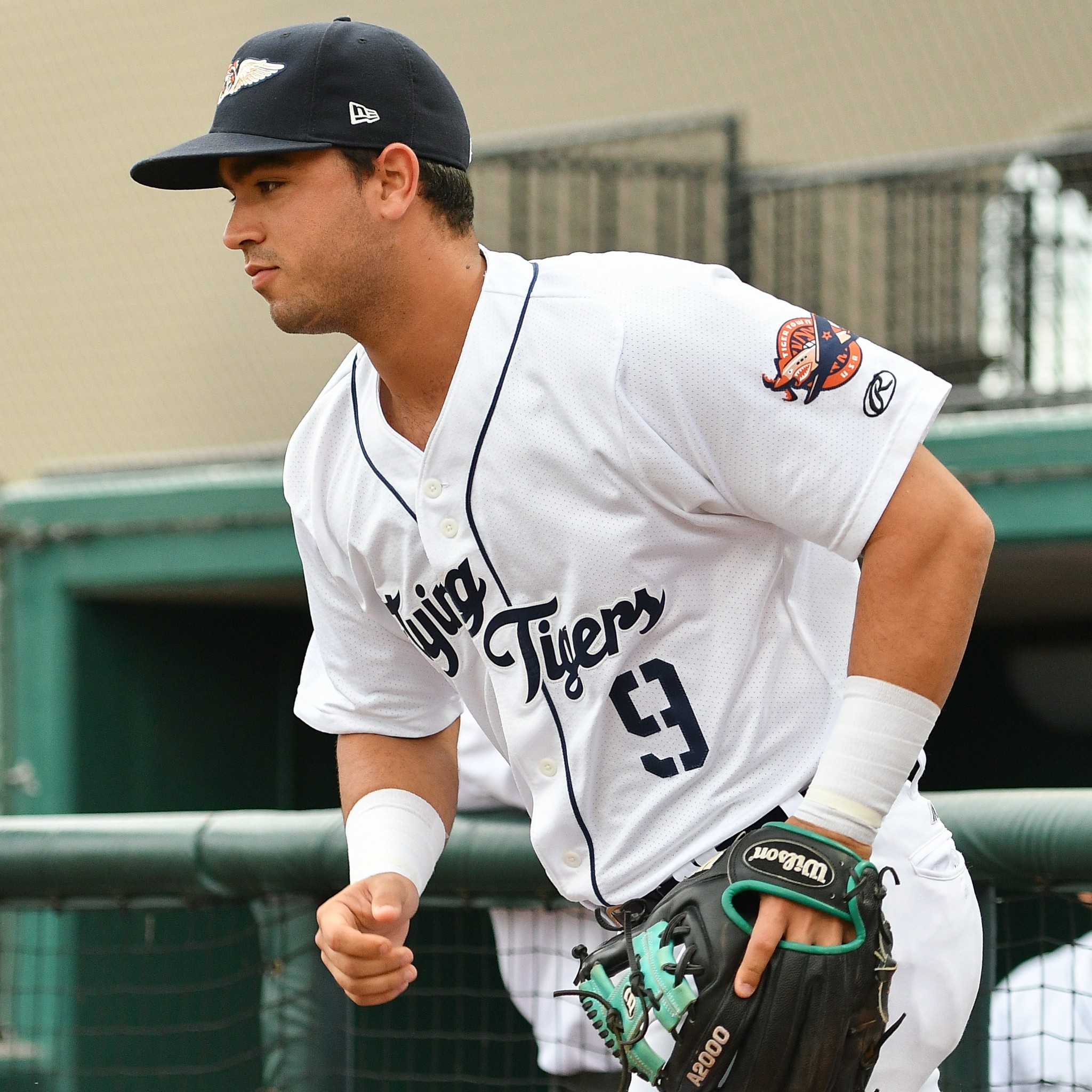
[595,761,921,937]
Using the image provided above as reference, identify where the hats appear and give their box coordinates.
[131,16,471,191]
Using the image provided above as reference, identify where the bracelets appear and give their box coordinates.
[346,787,449,898]
[791,675,942,846]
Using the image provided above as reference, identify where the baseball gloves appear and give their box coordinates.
[547,819,912,1092]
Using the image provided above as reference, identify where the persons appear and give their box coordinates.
[130,17,995,1092]
[988,892,1092,1092]
